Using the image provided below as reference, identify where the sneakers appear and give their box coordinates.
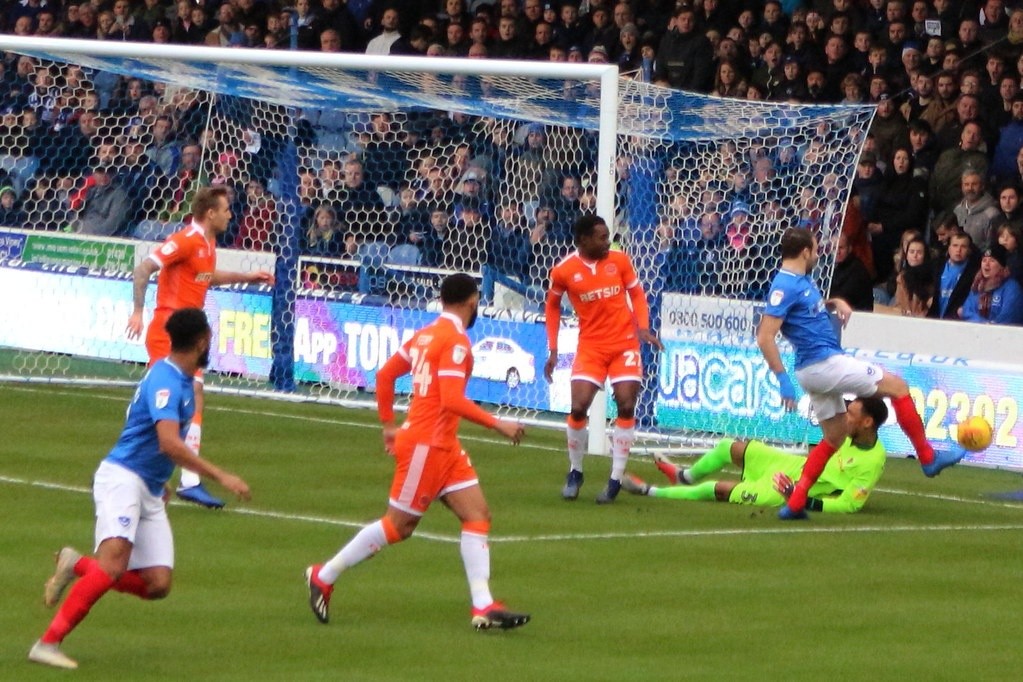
[471,603,531,630]
[561,469,584,498]
[596,478,620,503]
[921,446,966,479]
[45,545,78,607]
[778,504,807,520]
[306,564,334,624]
[621,473,649,496]
[176,482,225,510]
[28,638,80,668]
[655,451,686,484]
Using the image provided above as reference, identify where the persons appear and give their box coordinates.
[757,225,970,522]
[540,212,668,507]
[617,388,891,513]
[23,304,257,671]
[0,0,1023,333]
[304,272,533,635]
[125,183,280,513]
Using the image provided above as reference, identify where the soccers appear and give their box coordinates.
[957,416,993,452]
